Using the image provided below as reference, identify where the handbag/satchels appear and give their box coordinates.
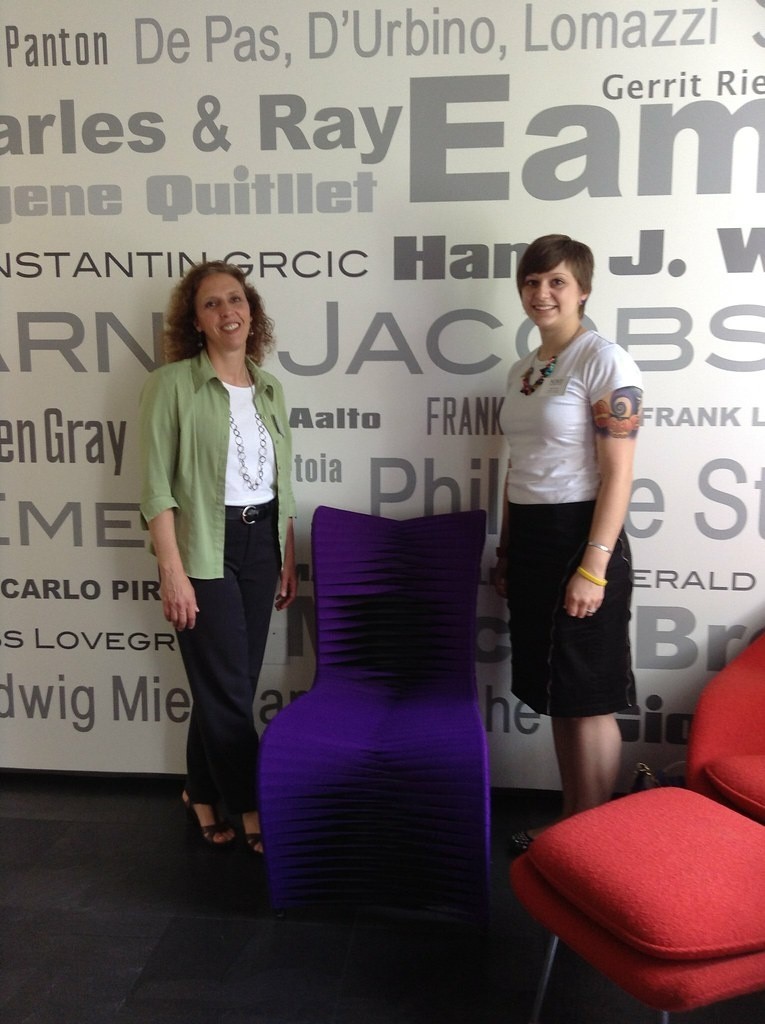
[629,761,687,792]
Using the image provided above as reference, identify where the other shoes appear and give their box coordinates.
[508,833,531,854]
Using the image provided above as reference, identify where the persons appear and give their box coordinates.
[490,234,644,850]
[138,260,297,856]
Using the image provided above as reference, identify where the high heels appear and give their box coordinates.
[242,813,266,857]
[182,789,236,851]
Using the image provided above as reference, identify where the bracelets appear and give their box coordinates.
[578,567,608,586]
[588,542,613,555]
[495,547,509,557]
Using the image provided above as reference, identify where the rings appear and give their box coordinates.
[587,610,595,614]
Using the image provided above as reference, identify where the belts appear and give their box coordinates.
[223,505,278,525]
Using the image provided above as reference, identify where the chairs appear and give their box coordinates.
[504,633,765,1024]
[255,508,491,908]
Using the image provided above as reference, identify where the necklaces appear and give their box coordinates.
[229,363,268,491]
[519,327,581,396]
